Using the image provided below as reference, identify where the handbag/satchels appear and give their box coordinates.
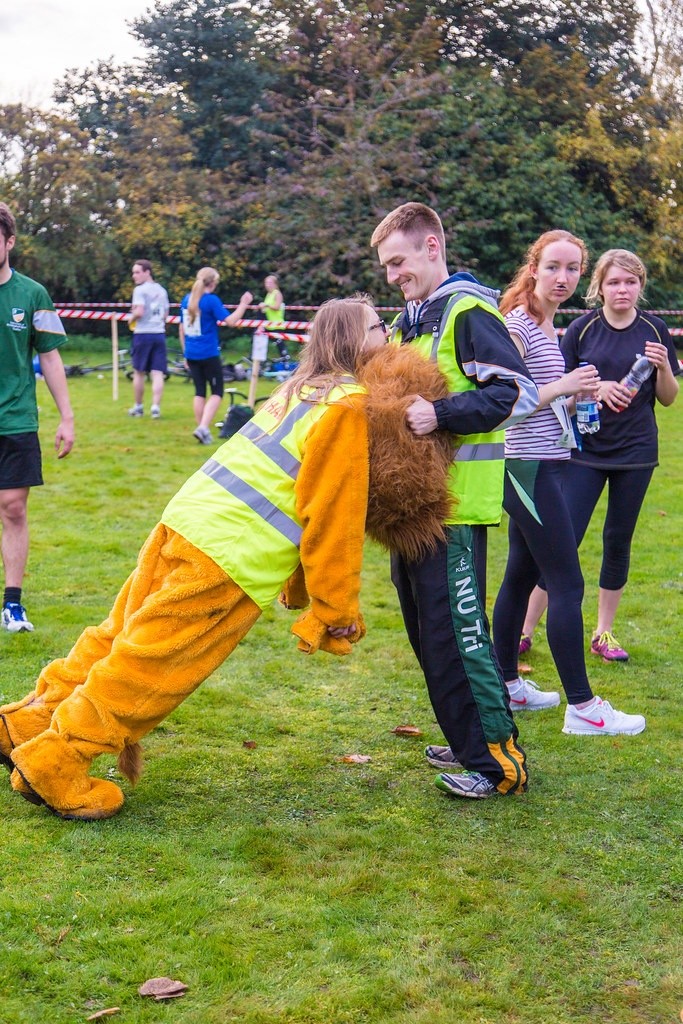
[217,405,255,438]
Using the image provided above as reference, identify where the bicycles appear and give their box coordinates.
[61,346,253,387]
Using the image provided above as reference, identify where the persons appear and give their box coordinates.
[0,295,392,818]
[179,267,253,444]
[126,258,169,419]
[365,202,540,803]
[257,274,291,362]
[515,248,683,664]
[0,203,78,633]
[492,226,649,736]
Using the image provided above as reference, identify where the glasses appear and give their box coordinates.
[366,317,387,333]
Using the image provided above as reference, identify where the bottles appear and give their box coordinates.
[576,361,600,435]
[611,354,654,412]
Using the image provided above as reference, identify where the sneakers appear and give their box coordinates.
[193,426,212,444]
[435,769,501,798]
[150,407,160,418]
[2,601,35,634]
[510,676,561,711]
[518,633,532,654]
[128,406,144,417]
[561,696,645,735]
[591,630,630,662]
[425,744,463,769]
